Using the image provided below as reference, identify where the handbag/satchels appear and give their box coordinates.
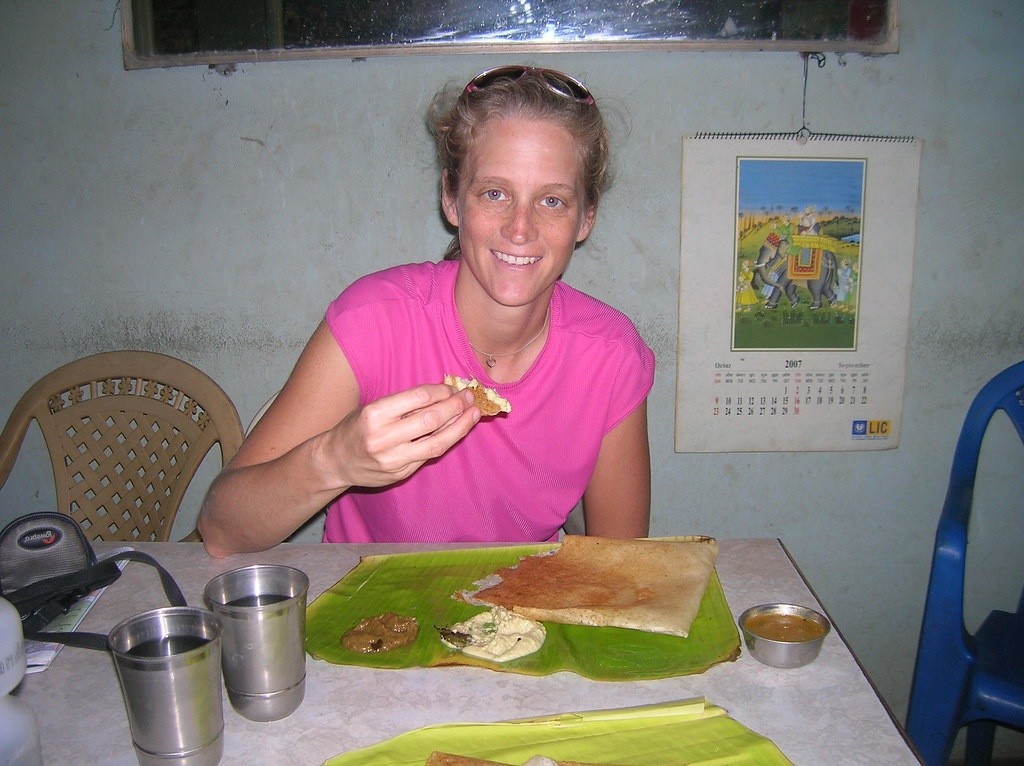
[0,511,98,614]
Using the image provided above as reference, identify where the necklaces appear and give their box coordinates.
[469,304,550,367]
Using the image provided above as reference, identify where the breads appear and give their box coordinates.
[443,375,512,416]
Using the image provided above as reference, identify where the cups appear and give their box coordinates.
[110,607,225,766]
[205,564,309,722]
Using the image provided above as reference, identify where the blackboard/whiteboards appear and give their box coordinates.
[117,0,906,74]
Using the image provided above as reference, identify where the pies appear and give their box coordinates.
[449,533,719,638]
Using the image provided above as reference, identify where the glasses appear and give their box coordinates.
[460,66,595,105]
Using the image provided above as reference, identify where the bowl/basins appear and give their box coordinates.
[738,603,831,669]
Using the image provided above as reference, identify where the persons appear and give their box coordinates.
[195,66,656,560]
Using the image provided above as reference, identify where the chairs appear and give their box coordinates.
[0,349,246,544]
[899,361,1024,766]
[0,534,924,766]
